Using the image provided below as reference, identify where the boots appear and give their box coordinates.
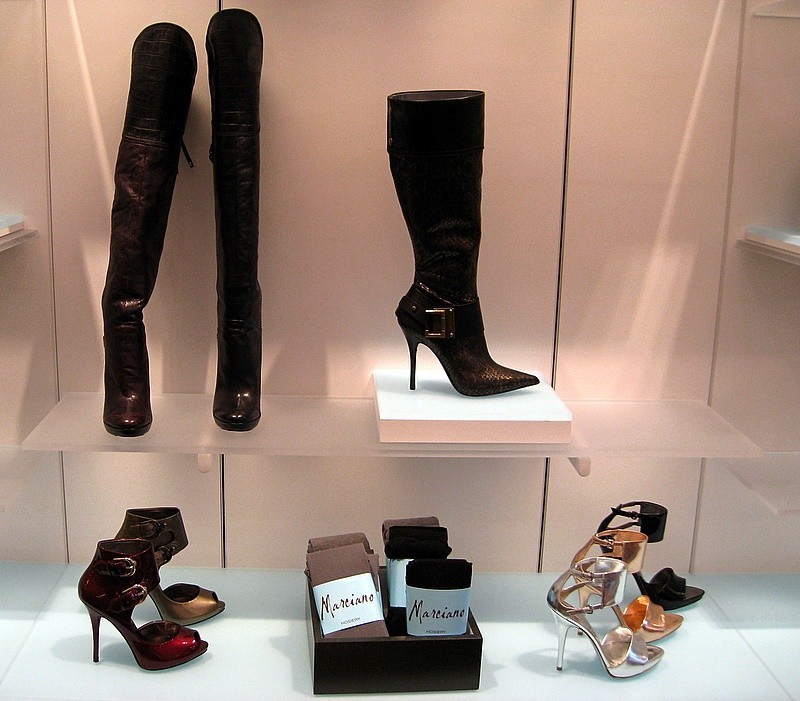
[204,7,265,433]
[385,88,542,397]
[98,21,198,438]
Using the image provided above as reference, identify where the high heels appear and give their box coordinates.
[546,556,665,679]
[569,528,685,644]
[77,538,210,671]
[595,500,706,612]
[115,505,226,627]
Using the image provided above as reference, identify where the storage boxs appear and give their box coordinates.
[309,566,483,694]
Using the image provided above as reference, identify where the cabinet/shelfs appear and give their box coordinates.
[1,0,800,701]
